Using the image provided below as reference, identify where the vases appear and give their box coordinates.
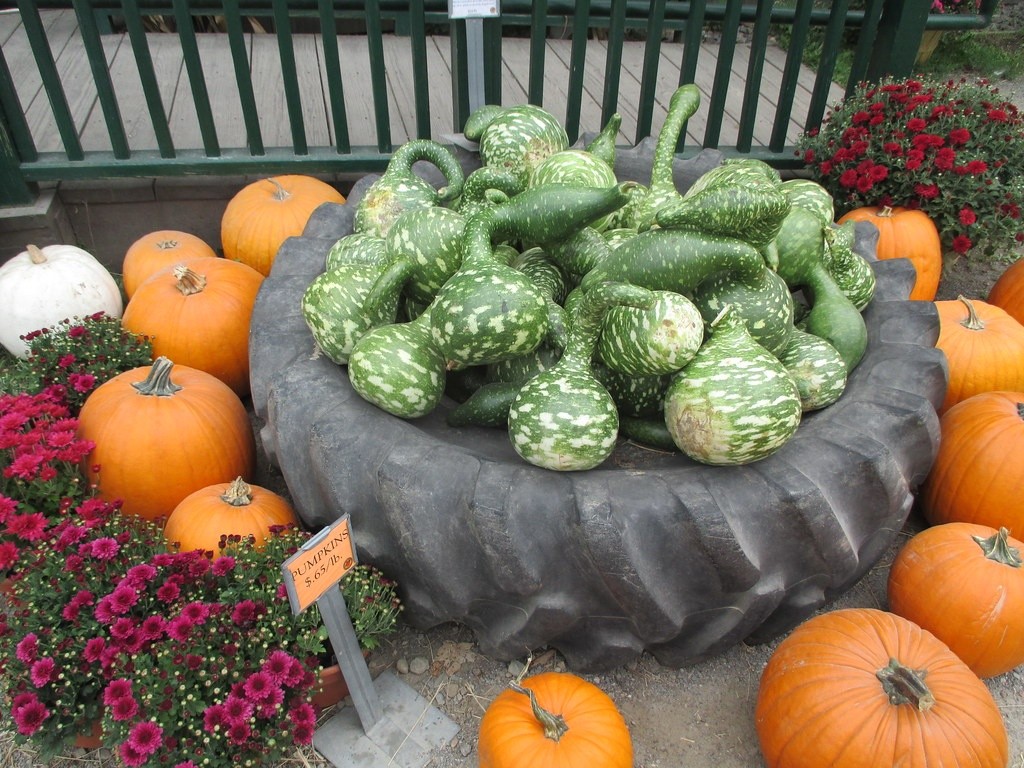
[290,642,372,709]
[912,29,943,66]
[0,571,41,606]
[63,691,116,745]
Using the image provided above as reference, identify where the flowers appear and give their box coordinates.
[0,313,405,767]
[929,0,982,14]
[794,71,1023,267]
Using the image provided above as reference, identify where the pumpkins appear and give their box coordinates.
[931,294,1024,417]
[985,257,1024,326]
[75,354,256,529]
[120,257,267,396]
[122,230,217,301]
[163,475,299,563]
[834,205,943,301]
[887,522,1024,678]
[920,391,1024,546]
[757,607,1009,768]
[478,658,634,768]
[0,244,123,361]
[221,174,346,277]
